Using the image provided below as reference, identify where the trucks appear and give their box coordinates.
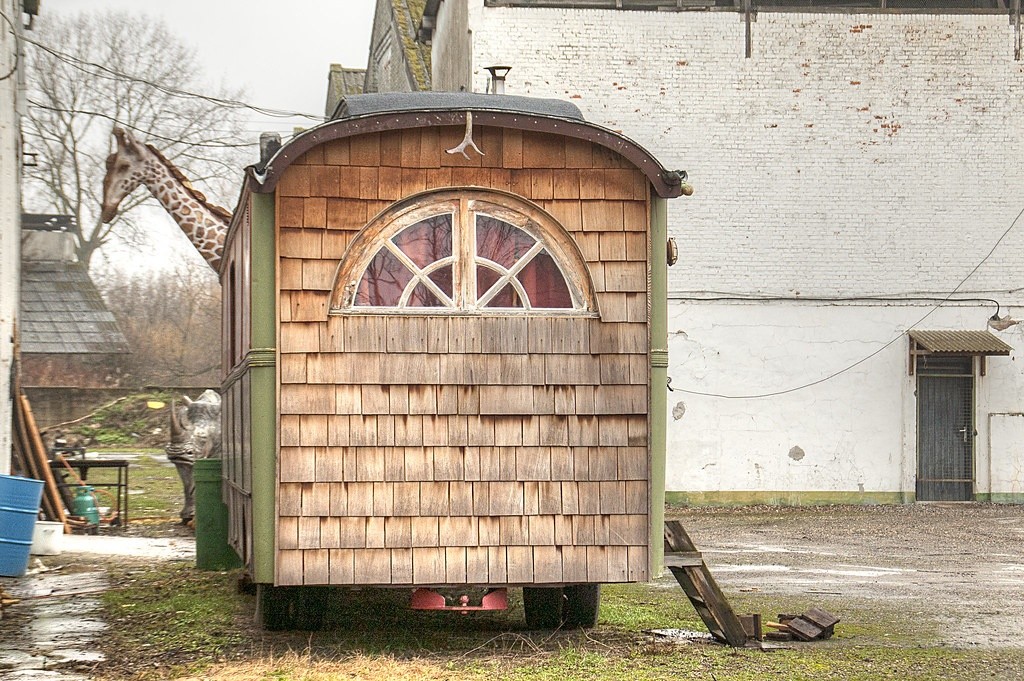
[218,91,694,635]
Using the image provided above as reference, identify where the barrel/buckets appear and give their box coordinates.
[1,475,46,577]
[194,460,241,570]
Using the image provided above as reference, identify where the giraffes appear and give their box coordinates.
[100,126,233,276]
